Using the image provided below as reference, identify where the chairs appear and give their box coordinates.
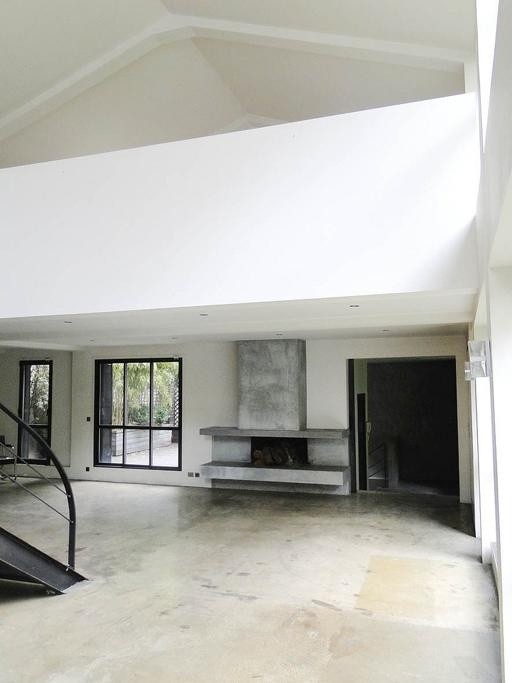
[0,433,18,485]
